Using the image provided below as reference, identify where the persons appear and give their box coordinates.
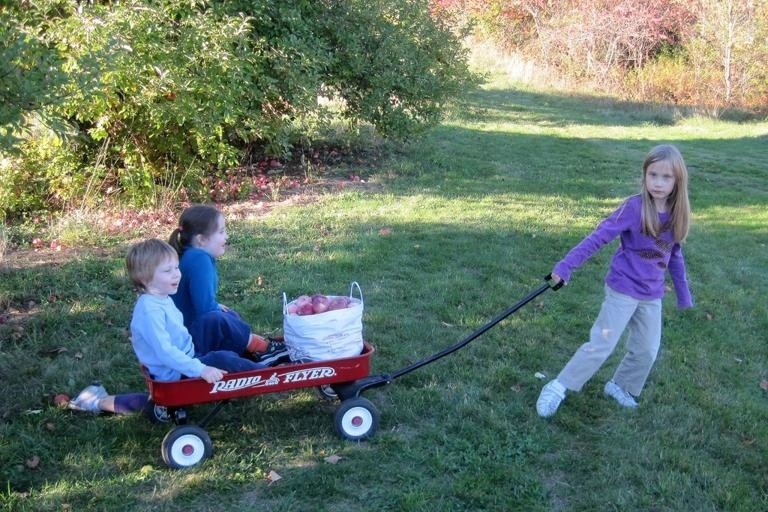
[67,205,241,416]
[536,143,693,418]
[126,238,289,384]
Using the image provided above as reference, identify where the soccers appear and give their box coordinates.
[287,294,360,315]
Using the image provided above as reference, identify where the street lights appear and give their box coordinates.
[281,281,363,363]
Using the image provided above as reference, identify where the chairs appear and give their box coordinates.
[69,385,108,415]
[604,379,639,407]
[536,379,566,418]
